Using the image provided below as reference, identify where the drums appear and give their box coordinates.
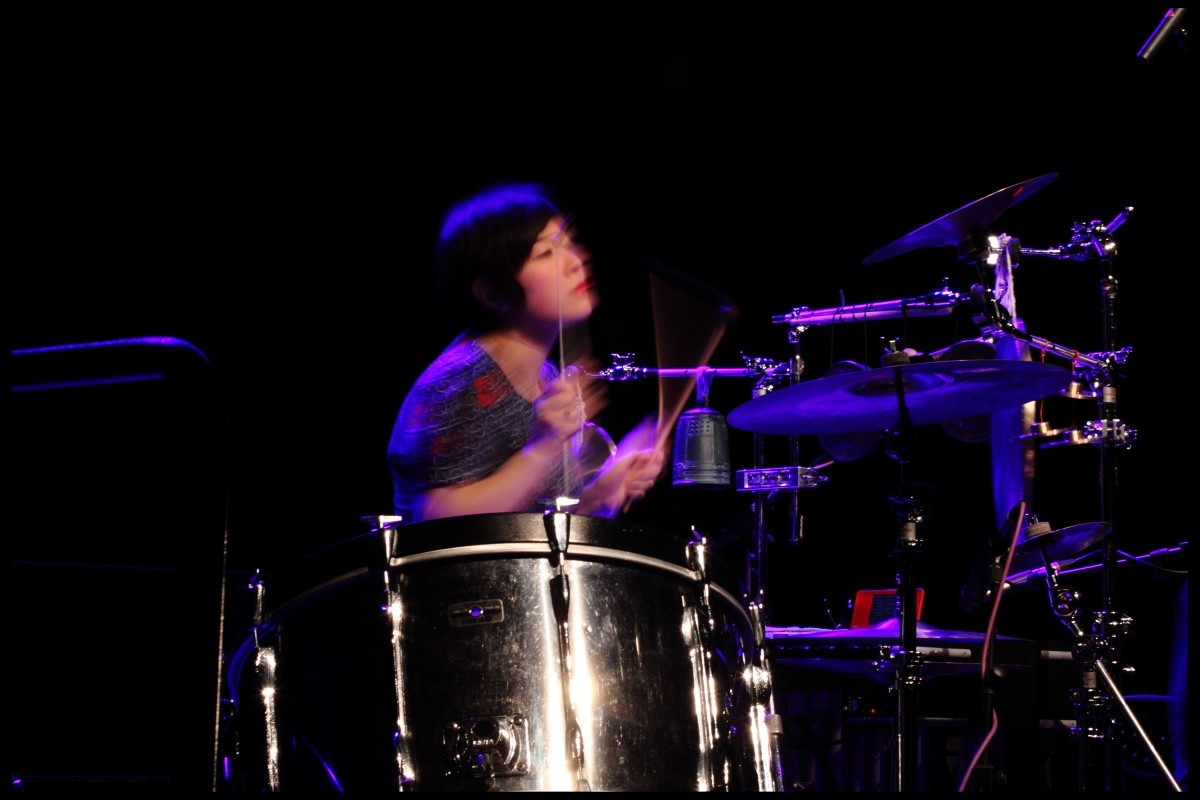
[209,498,787,799]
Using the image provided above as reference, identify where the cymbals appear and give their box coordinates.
[765,617,1033,647]
[1004,521,1114,576]
[859,172,1062,267]
[726,359,1074,433]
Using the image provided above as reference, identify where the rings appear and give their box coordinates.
[564,411,570,419]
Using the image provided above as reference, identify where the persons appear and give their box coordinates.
[386,186,667,524]
